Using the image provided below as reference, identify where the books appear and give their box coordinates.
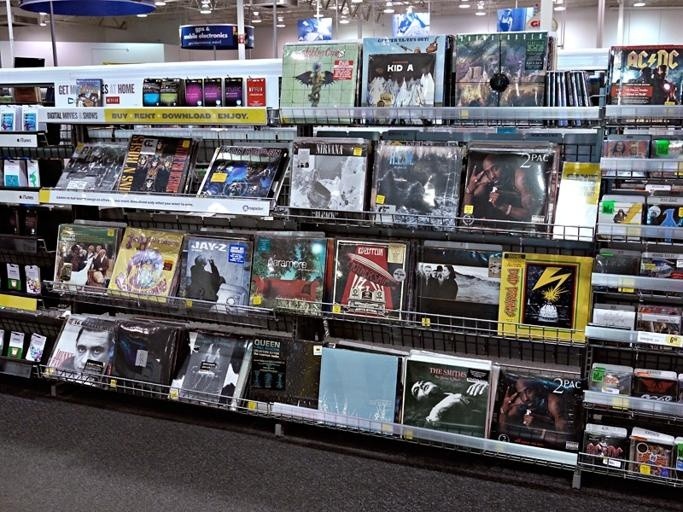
[0,30,683,485]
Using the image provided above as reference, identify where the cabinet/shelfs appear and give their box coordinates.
[0,57,682,494]
